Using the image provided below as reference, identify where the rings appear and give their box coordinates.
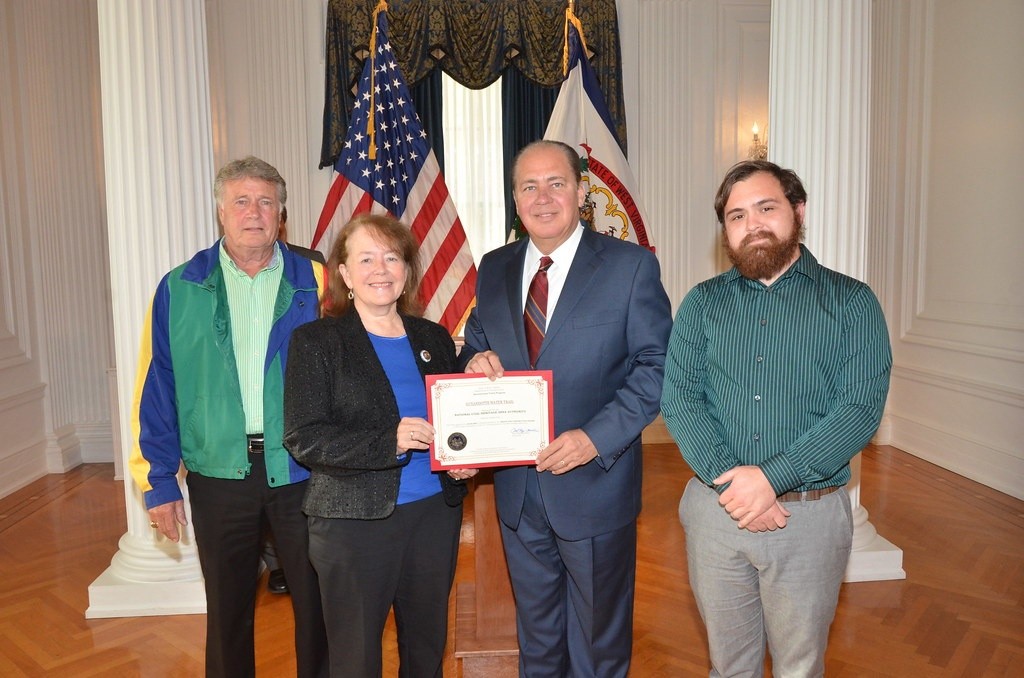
[409,431,415,440]
[560,459,568,467]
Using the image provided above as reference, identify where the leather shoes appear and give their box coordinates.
[268,557,288,594]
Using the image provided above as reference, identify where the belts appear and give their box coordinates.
[248,437,264,453]
[777,486,839,502]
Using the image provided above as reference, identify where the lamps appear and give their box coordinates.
[749,121,768,160]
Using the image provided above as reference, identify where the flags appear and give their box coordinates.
[503,0,657,263]
[310,1,481,360]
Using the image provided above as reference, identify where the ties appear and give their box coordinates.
[524,256,553,370]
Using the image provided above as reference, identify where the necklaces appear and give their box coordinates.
[150,521,159,529]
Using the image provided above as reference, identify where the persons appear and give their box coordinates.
[457,141,674,678]
[125,157,335,677]
[281,210,482,678]
[658,159,894,677]
